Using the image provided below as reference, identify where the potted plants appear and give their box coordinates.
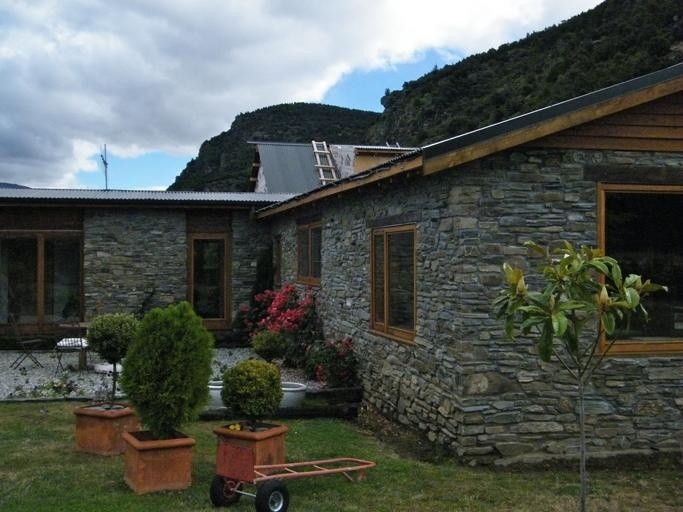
[117,302,216,495]
[74,311,142,457]
[213,358,288,481]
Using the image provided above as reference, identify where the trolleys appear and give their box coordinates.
[210,456,375,512]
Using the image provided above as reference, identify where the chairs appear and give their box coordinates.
[9,311,45,369]
[54,321,93,376]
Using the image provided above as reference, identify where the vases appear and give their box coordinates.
[280,382,308,407]
[208,381,225,408]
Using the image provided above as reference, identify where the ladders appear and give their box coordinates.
[311,140,338,185]
